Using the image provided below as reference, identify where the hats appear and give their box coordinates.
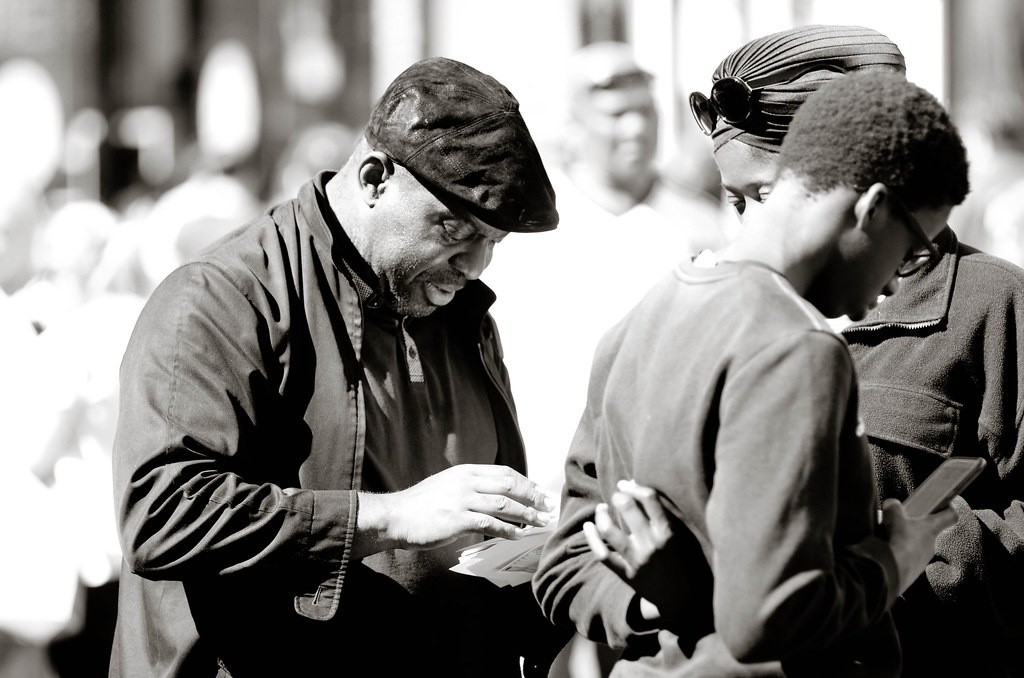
[711,24,906,155]
[366,56,559,233]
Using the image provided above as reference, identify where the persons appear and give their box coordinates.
[107,56,559,678]
[531,23,1024,678]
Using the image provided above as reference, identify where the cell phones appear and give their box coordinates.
[875,457,986,544]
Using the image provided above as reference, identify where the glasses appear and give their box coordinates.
[587,69,653,91]
[690,61,846,135]
[854,183,940,277]
